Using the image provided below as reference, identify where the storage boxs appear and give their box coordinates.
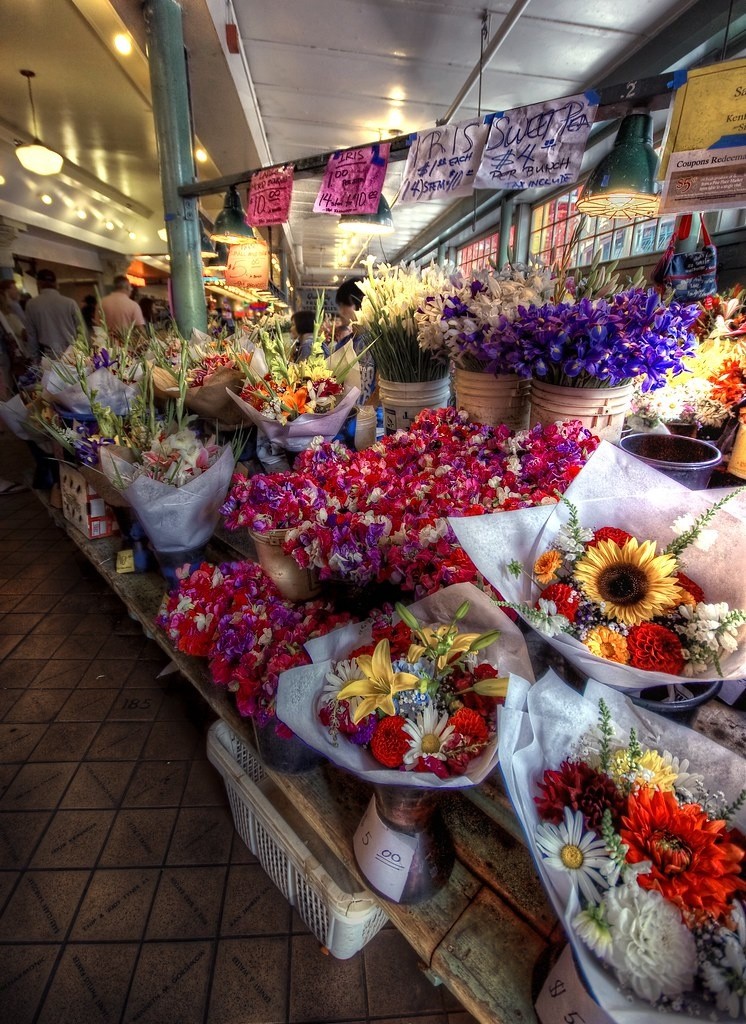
[205,719,391,960]
[60,456,112,538]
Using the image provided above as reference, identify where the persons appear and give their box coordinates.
[0,269,86,369]
[93,275,146,334]
[138,298,158,325]
[334,277,384,420]
[290,310,331,364]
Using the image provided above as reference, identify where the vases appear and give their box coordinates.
[147,540,204,599]
[531,938,618,1024]
[351,783,456,906]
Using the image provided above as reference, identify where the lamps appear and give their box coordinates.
[574,110,661,219]
[338,191,394,236]
[126,261,146,287]
[184,46,219,259]
[204,240,230,271]
[14,69,64,176]
[209,190,257,244]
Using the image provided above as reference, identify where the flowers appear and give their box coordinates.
[32,215,746,1024]
